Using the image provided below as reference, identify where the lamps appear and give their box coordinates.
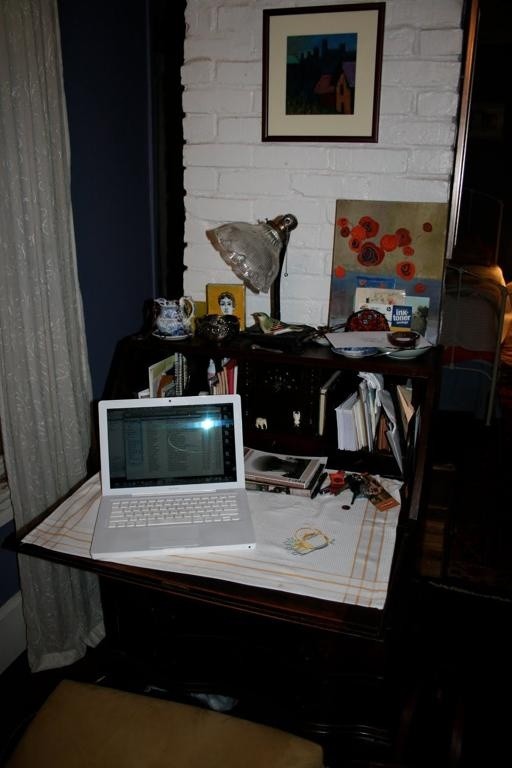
[205,213,318,352]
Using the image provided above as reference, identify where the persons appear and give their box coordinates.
[218,291,236,315]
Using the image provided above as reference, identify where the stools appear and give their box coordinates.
[4,677,324,768]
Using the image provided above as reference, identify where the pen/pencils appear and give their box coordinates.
[310,472,328,500]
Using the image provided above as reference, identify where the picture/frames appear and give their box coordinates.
[260,1,388,144]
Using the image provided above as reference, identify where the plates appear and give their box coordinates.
[331,348,378,358]
[152,329,193,340]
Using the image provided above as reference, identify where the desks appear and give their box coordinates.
[1,446,409,644]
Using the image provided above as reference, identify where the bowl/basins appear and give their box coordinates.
[375,346,431,359]
[390,331,419,346]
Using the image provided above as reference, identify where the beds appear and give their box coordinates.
[442,257,512,426]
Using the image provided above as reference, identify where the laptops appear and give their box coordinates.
[89,394,258,558]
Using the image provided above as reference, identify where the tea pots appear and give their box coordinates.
[152,296,196,335]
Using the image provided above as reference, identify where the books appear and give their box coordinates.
[214,360,238,395]
[149,355,175,398]
[244,457,329,500]
[319,370,341,436]
[179,353,182,396]
[175,352,178,396]
[335,371,421,475]
[184,356,187,390]
[238,447,321,489]
[161,382,175,397]
[207,284,246,332]
